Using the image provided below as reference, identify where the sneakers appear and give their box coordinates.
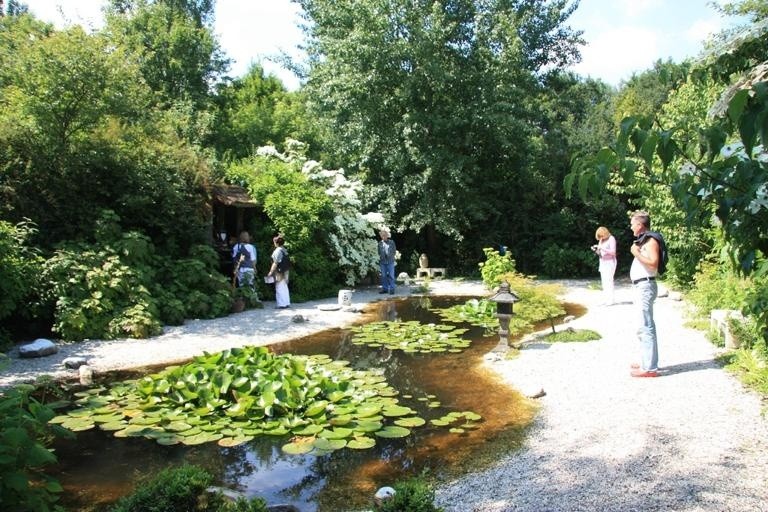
[629,362,658,378]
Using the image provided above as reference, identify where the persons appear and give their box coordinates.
[590,226,617,306]
[266,236,292,309]
[377,230,397,295]
[628,210,659,378]
[231,230,262,304]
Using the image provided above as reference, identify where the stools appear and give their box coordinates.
[417,268,449,278]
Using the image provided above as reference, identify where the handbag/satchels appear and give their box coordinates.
[275,249,290,274]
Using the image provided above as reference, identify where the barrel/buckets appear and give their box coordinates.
[338,289,352,306]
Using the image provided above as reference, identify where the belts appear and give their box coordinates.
[633,276,656,285]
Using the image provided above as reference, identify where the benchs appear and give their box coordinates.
[710,309,743,350]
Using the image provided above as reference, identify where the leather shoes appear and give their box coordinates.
[378,287,394,295]
